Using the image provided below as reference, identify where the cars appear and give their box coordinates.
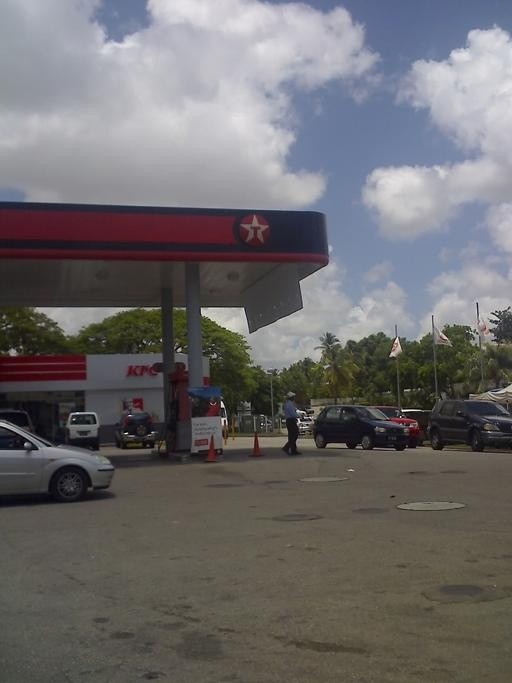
[296,399,512,451]
[0,409,114,502]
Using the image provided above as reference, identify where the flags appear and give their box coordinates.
[389,336,402,357]
[434,326,453,347]
[475,319,492,337]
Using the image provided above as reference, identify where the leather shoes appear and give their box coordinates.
[281,447,290,455]
[290,450,303,456]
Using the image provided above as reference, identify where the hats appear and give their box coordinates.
[287,391,296,398]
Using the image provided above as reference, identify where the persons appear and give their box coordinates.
[281,391,307,455]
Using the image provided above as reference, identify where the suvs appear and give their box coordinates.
[115,413,156,449]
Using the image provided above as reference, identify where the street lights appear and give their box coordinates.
[266,367,278,433]
[313,344,333,351]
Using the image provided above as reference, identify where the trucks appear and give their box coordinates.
[221,400,229,439]
